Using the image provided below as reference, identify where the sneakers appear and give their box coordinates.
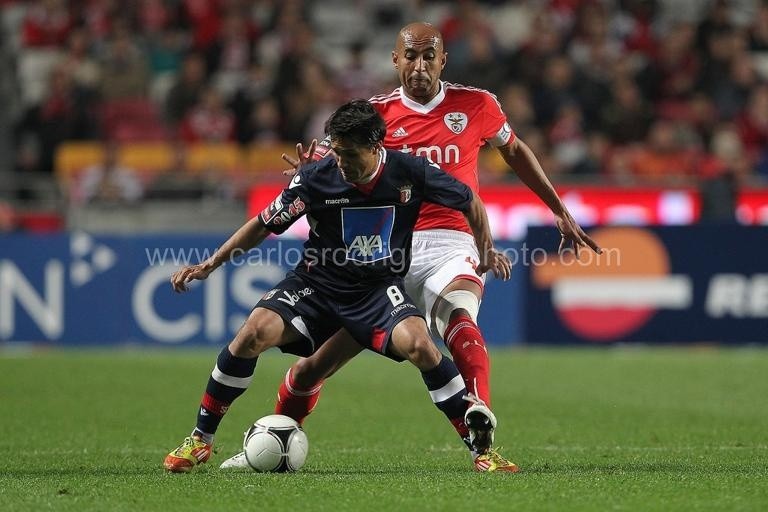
[161,429,213,473]
[463,401,497,455]
[470,448,519,475]
[218,451,252,470]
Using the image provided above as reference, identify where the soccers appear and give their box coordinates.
[244,414,308,472]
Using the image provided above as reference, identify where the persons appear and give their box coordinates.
[217,21,604,469]
[161,98,521,473]
